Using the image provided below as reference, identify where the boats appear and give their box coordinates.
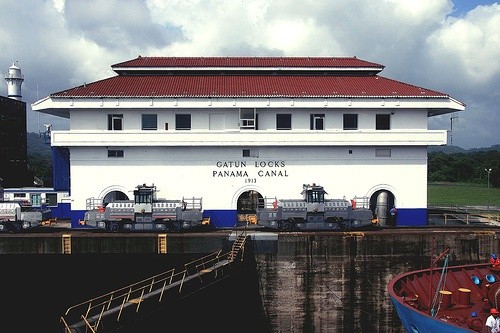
[388,249,500,333]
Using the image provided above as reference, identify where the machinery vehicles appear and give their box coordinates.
[253,185,380,233]
[0,196,58,232]
[84,184,212,233]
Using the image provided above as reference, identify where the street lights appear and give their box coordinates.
[484,168,492,214]
